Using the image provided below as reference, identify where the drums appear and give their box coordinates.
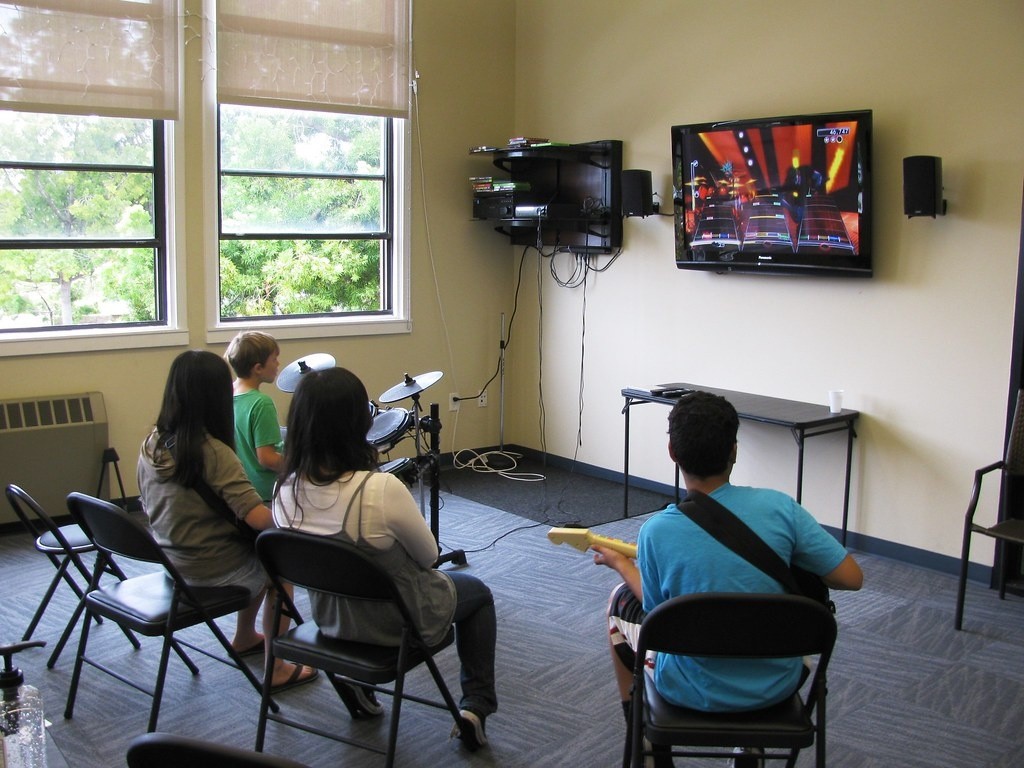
[378,457,412,475]
[366,408,412,446]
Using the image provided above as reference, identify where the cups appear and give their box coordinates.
[829,392,843,413]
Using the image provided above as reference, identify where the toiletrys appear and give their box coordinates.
[0,640,49,768]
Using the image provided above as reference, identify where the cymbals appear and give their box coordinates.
[378,370,444,404]
[276,353,336,393]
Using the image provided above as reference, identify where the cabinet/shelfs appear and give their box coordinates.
[467,140,622,254]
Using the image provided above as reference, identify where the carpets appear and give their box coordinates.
[422,453,681,528]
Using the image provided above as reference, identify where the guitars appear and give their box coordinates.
[545,526,831,609]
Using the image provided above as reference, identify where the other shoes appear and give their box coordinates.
[640,736,654,768]
[727,741,764,768]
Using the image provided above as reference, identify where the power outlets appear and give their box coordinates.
[449,392,461,411]
[479,390,487,407]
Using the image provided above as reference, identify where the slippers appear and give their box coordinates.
[228,637,265,658]
[268,661,319,694]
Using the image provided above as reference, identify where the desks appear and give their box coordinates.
[622,384,860,549]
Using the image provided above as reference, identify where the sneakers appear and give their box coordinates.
[335,679,384,716]
[448,707,488,747]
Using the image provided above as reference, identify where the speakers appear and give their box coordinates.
[621,170,654,219]
[902,154,944,220]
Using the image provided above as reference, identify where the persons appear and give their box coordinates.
[272,367,497,744]
[225,331,285,506]
[135,350,316,695]
[590,391,863,768]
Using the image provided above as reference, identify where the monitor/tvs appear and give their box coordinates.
[671,110,874,278]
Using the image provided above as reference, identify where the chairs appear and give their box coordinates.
[955,391,1024,629]
[254,527,478,768]
[126,733,309,768]
[63,492,281,733]
[5,484,141,667]
[623,592,838,768]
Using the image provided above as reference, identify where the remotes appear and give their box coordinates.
[651,388,696,398]
